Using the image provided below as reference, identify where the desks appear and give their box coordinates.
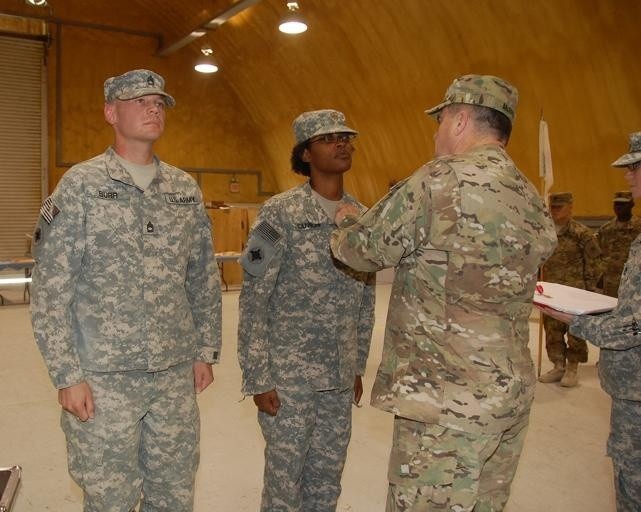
[0,257,35,279]
[214,253,242,292]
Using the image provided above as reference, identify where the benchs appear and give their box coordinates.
[0,277,32,305]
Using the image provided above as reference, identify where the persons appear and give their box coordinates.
[538,131,641,512]
[597,191,641,296]
[331,75,558,512]
[31,72,222,512]
[237,111,376,512]
[539,192,601,388]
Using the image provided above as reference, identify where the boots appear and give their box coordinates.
[536,356,566,385]
[560,359,579,388]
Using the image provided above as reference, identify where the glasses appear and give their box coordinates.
[626,161,641,171]
[307,132,357,144]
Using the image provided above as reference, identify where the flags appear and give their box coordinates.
[539,119,553,195]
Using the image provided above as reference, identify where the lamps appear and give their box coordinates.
[279,2,307,35]
[194,45,219,74]
[25,0,47,8]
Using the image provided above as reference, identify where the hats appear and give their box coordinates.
[546,191,574,209]
[609,130,641,167]
[101,67,177,110]
[424,72,521,122]
[292,107,359,147]
[610,190,635,204]
